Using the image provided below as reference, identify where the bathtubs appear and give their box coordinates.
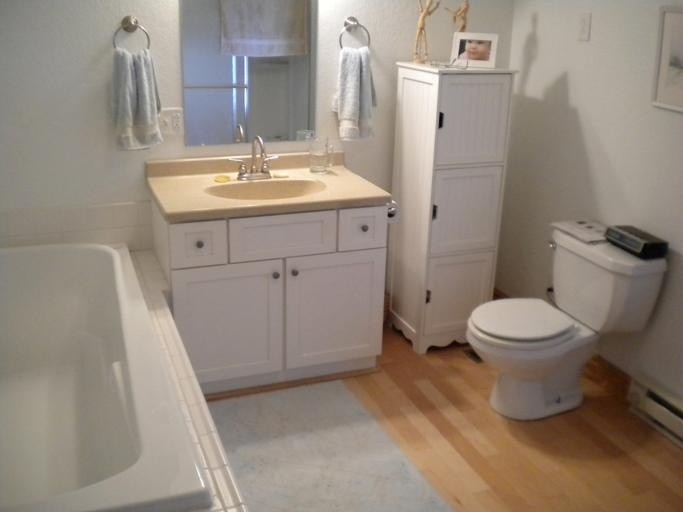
[1,241,214,512]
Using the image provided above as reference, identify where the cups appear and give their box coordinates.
[295,130,314,142]
[308,137,334,173]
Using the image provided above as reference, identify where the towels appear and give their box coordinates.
[331,46,378,143]
[220,1,311,57]
[112,46,165,152]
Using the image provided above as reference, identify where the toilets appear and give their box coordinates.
[465,224,667,421]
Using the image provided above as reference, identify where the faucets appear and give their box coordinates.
[250,135,266,173]
[237,124,245,142]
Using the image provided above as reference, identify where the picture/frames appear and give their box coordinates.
[449,31,499,69]
[650,4,682,114]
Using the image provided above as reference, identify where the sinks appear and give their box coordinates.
[203,179,326,200]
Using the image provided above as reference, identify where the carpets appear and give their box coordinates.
[206,379,455,512]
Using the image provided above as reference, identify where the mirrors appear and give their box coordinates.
[178,0,318,146]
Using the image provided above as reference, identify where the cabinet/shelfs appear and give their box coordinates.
[150,196,390,396]
[386,61,518,356]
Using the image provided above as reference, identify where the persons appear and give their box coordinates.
[458,39,492,61]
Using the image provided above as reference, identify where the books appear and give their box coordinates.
[549,217,608,243]
